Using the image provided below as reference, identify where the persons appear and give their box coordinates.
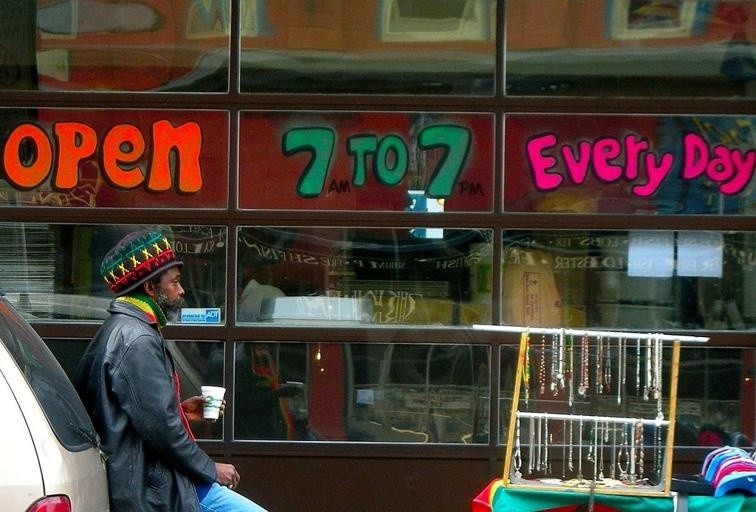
[206,256,290,441]
[71,227,273,511]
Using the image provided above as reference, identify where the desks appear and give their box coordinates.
[496,478,756,511]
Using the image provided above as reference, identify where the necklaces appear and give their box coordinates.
[510,326,666,484]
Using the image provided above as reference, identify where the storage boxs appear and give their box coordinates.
[595,234,682,333]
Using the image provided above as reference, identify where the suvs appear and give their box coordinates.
[1,299,110,511]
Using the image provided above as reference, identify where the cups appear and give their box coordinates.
[199,386,226,422]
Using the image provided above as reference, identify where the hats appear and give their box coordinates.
[99,229,184,299]
